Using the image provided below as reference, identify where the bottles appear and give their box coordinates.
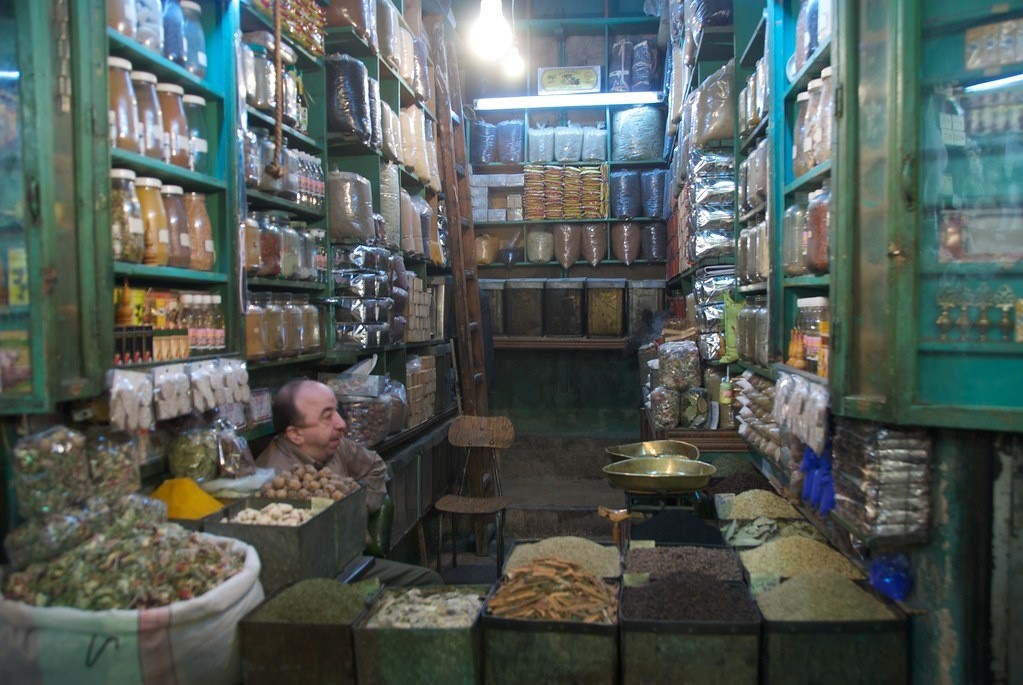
[244,38,327,361]
[736,295,831,379]
[105,0,228,367]
[737,0,831,280]
[719,376,738,430]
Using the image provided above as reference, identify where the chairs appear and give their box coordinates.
[436,416,515,579]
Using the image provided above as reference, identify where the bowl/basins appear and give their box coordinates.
[604,440,700,463]
[602,457,717,494]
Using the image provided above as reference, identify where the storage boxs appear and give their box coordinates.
[626,279,666,335]
[506,279,547,337]
[479,279,508,336]
[538,65,602,95]
[545,278,588,335]
[167,487,912,684]
[428,275,454,342]
[585,279,629,336]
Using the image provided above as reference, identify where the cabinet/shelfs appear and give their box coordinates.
[665,0,1022,618]
[1,0,465,565]
[457,1,671,350]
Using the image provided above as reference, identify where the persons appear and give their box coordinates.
[254,380,443,586]
